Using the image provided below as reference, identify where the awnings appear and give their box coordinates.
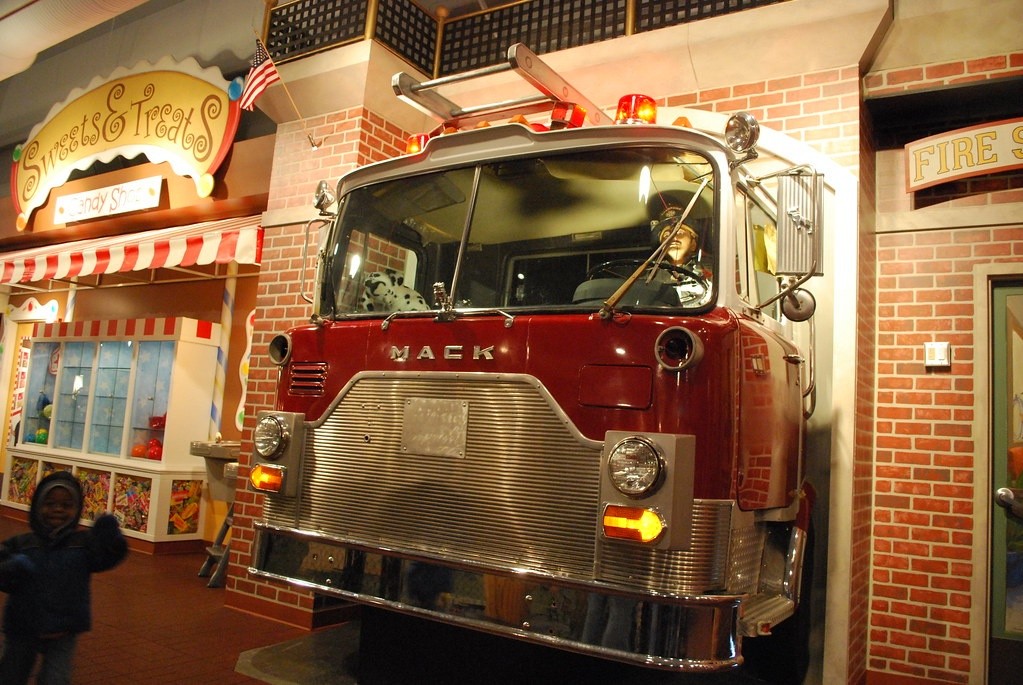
[1,210,265,295]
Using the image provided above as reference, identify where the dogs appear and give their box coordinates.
[360,268,431,313]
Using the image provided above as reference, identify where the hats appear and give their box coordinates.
[650,201,699,249]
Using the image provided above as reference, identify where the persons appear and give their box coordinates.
[0,470,131,685]
[650,204,713,280]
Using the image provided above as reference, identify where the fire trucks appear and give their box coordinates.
[243,42,826,683]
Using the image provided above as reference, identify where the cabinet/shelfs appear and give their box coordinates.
[0,335,222,543]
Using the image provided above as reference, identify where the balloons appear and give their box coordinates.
[146,438,162,452]
[25,432,36,442]
[35,433,49,444]
[145,446,162,460]
[35,429,49,434]
[131,444,148,458]
